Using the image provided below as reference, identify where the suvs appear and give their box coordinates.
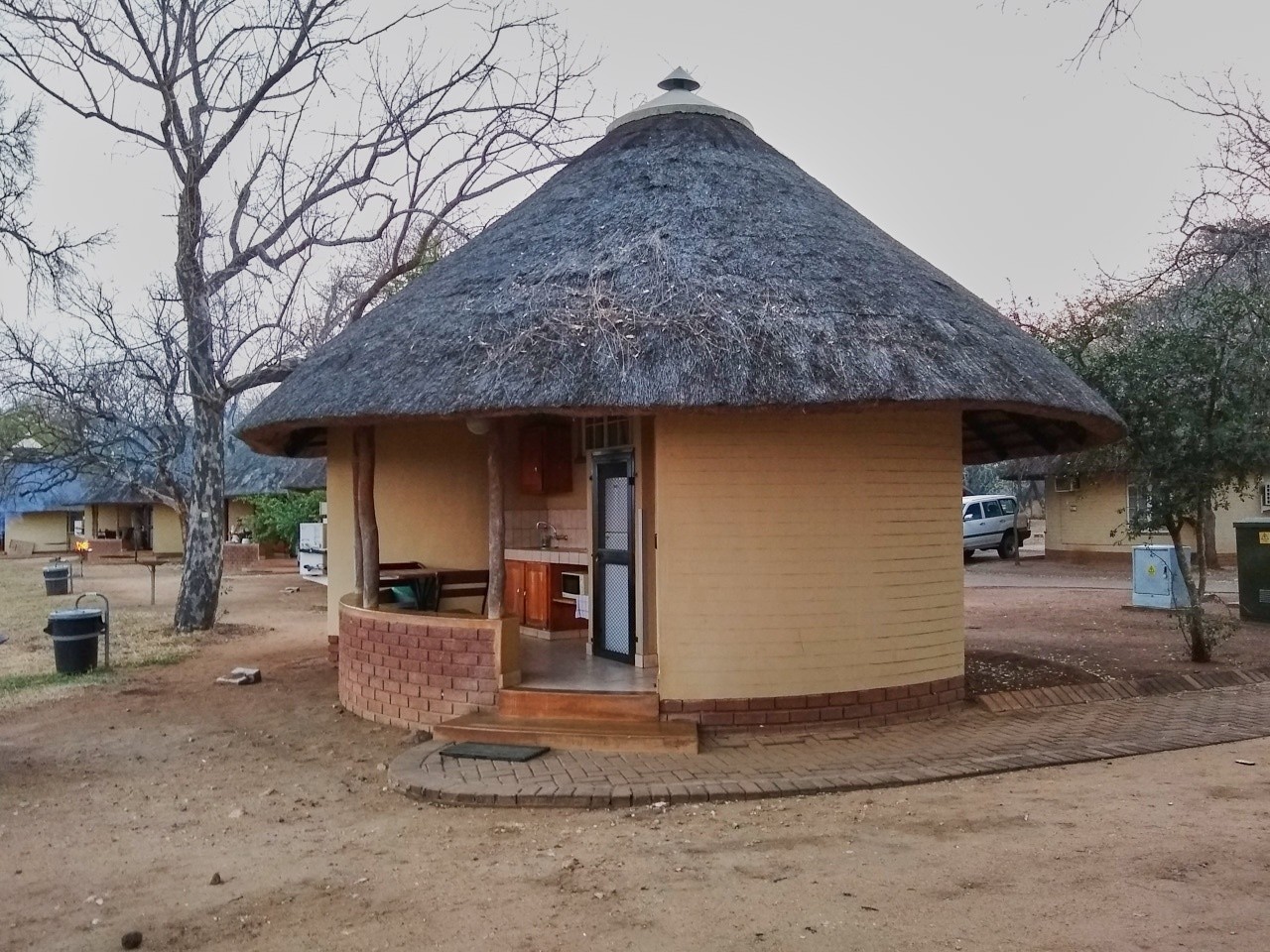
[962,494,1032,560]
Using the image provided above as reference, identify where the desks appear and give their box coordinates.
[375,568,478,611]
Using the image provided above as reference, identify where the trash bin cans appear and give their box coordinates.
[42,565,69,596]
[43,608,107,677]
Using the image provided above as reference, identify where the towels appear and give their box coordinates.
[575,594,589,619]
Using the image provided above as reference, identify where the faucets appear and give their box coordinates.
[536,520,557,538]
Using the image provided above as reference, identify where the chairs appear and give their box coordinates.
[431,569,489,616]
